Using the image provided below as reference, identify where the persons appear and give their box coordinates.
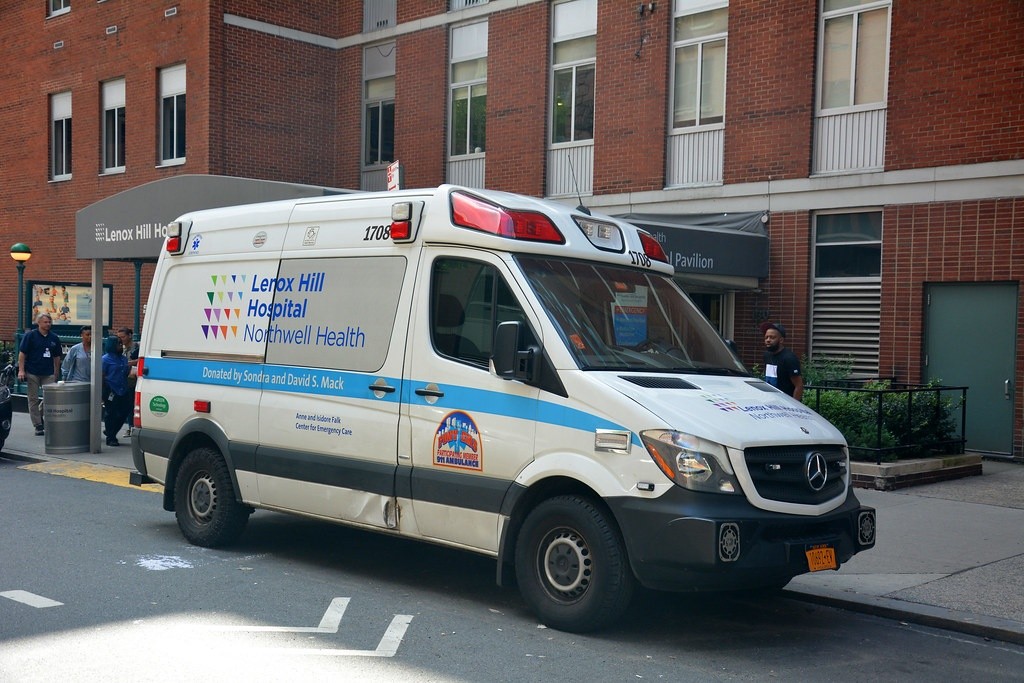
[61,326,91,382]
[17,315,62,436]
[762,323,803,402]
[32,286,72,325]
[102,335,129,447]
[117,327,140,438]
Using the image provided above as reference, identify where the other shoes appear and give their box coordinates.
[103,430,120,446]
[35,424,45,436]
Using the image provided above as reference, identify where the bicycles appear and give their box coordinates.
[0,358,15,393]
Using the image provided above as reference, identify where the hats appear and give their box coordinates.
[761,322,785,338]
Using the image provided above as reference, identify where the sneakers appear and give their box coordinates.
[122,430,131,438]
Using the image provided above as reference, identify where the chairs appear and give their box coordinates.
[437,294,480,358]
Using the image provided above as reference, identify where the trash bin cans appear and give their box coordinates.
[41,381,91,454]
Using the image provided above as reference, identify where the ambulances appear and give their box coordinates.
[129,183,878,636]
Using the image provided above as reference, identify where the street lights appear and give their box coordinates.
[10,242,32,393]
